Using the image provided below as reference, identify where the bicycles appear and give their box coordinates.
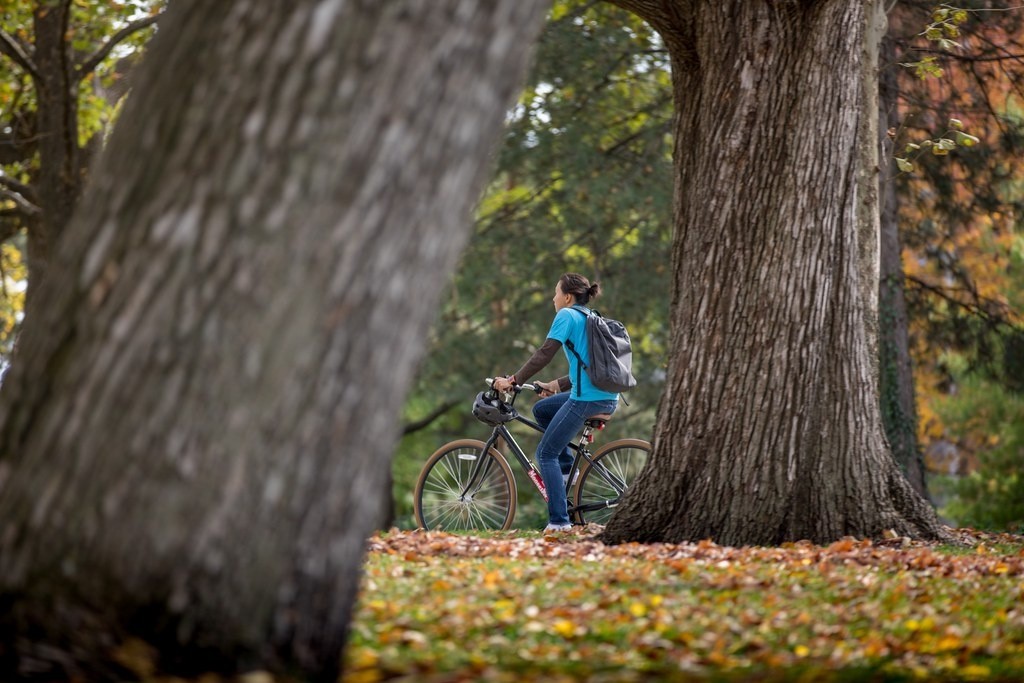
[414,377,652,530]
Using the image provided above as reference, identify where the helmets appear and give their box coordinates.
[472,392,519,426]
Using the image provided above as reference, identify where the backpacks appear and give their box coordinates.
[563,307,637,407]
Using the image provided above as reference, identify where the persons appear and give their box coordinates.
[493,273,621,535]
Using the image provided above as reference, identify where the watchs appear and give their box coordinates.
[507,376,516,386]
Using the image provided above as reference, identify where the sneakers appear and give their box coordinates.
[542,523,572,537]
[562,468,580,485]
